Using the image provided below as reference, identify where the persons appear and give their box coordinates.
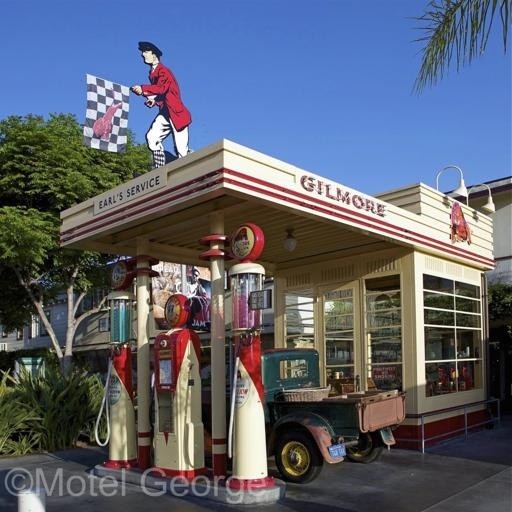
[186,266,208,297]
[128,40,193,170]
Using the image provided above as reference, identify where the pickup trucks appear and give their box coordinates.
[202,348,407,484]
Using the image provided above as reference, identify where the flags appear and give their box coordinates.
[80,73,131,156]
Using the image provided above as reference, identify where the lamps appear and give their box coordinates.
[283,229,297,252]
[436,165,496,213]
